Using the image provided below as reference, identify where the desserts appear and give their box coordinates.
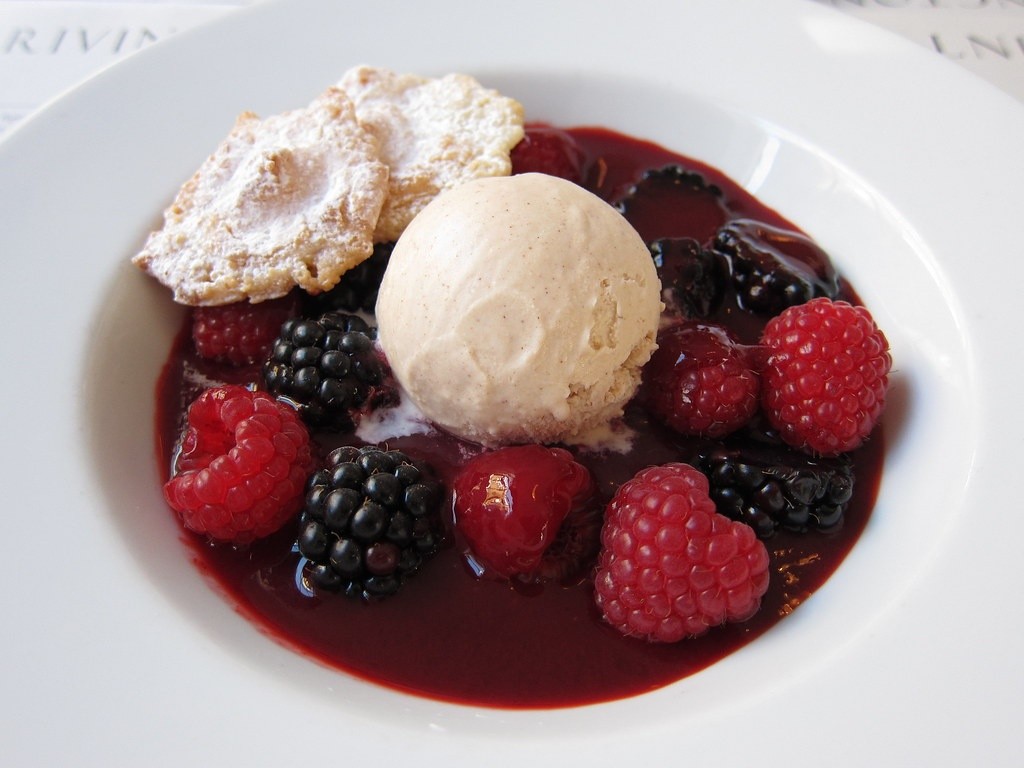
[374,170,662,447]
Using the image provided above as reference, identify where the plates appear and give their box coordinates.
[0,0,1023,768]
